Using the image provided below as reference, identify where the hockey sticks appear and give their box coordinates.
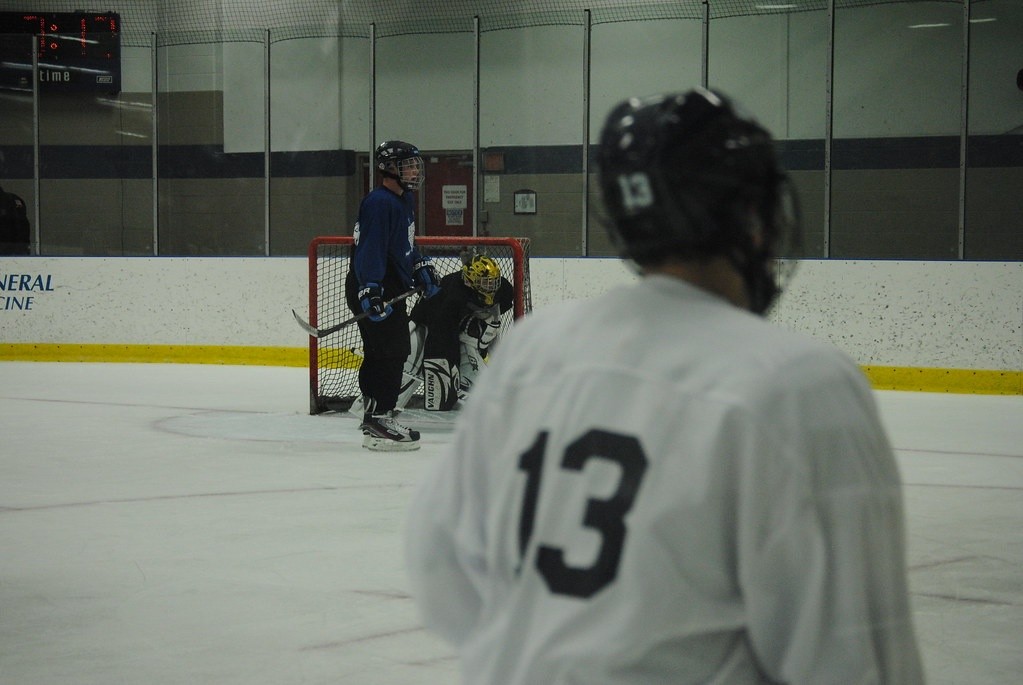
[292,282,427,338]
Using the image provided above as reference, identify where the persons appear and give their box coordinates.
[349,251,514,416]
[396,86,931,685]
[342,141,442,445]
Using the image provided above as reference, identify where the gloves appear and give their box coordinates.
[357,282,395,323]
[412,255,443,300]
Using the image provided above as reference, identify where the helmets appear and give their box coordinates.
[375,140,424,179]
[461,254,502,307]
[599,84,789,268]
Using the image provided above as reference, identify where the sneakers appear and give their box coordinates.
[359,408,421,452]
